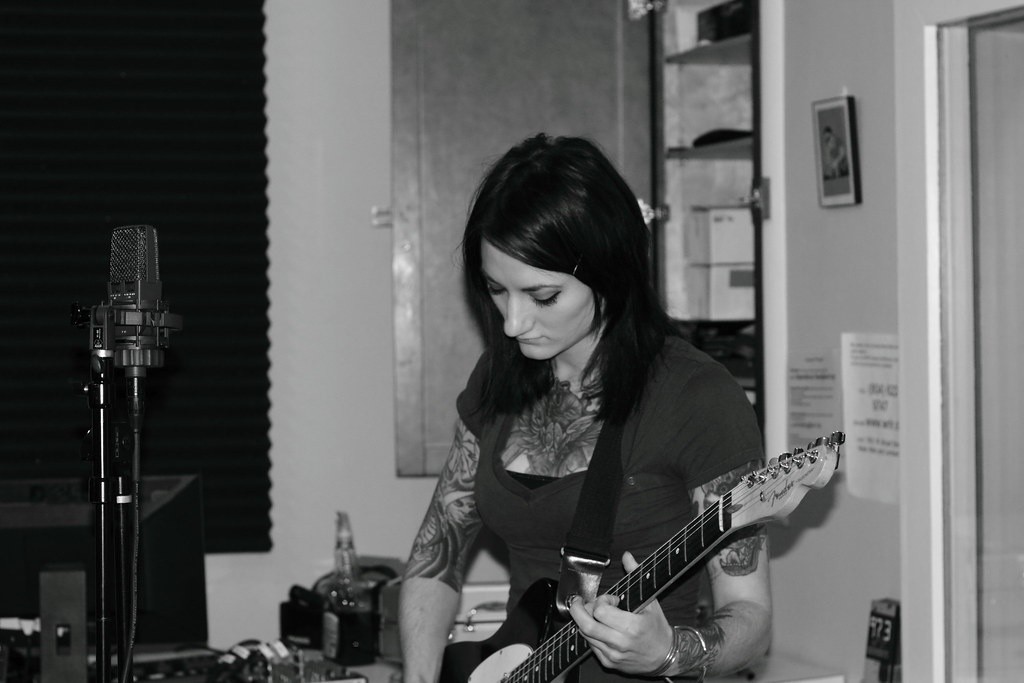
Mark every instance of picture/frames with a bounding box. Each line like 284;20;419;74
811;95;862;208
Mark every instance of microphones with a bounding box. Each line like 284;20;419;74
109;226;163;437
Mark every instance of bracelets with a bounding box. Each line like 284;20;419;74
649;625;707;683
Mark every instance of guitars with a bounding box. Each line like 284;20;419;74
436;428;848;682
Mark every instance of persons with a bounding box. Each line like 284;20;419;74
822;126;850;179
400;135;771;683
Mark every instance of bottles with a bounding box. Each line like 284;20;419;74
322;510;380;669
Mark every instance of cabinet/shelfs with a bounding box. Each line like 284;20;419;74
645;1;764;439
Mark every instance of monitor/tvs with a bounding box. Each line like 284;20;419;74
0;473;209;683
